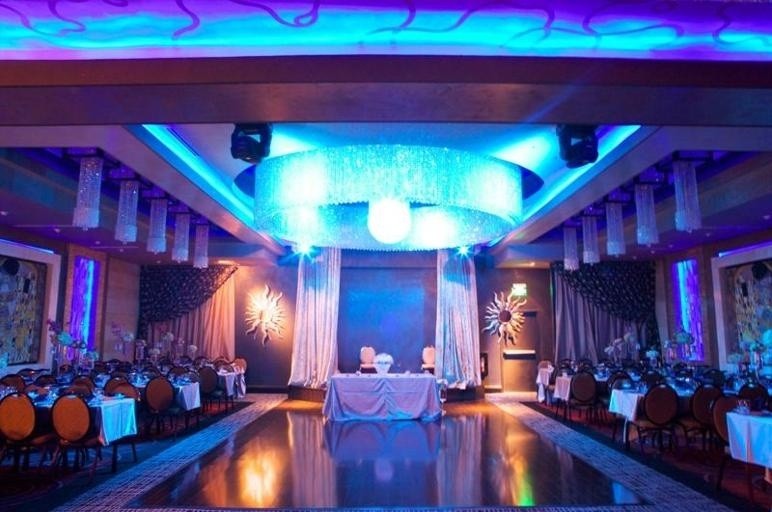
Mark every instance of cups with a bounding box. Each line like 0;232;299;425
734;399;751;415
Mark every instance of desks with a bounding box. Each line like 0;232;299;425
322;373;442;423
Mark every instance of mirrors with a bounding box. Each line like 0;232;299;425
244;284;286;347
480;288;527;345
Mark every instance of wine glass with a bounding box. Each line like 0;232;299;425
0;362;190;402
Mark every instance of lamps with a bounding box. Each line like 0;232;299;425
73;156;209;269
230;122;273;166
256;145;525;251
556;123;599;169
564;162;703;273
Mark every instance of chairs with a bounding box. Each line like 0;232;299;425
537;358;772;501
360;346;376;373
0;355;248;487
421;346;435;374
